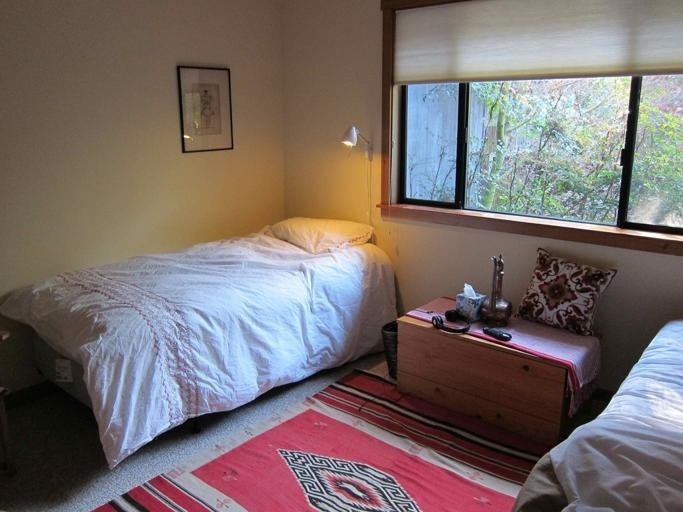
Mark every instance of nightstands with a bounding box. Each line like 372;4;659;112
397;300;603;446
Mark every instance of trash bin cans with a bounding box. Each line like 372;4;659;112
381;322;398;380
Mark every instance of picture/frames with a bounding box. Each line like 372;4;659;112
178;66;235;153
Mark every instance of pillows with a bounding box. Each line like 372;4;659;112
512;247;616;336
270;216;375;253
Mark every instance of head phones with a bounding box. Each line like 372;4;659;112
432;310;470;333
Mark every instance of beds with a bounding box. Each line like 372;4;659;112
2;219;395;469
526;321;682;512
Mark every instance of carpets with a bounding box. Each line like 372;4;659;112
95;371;545;512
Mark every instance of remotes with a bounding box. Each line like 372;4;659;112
483;326;512;341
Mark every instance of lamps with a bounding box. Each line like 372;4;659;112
340;124;375;161
